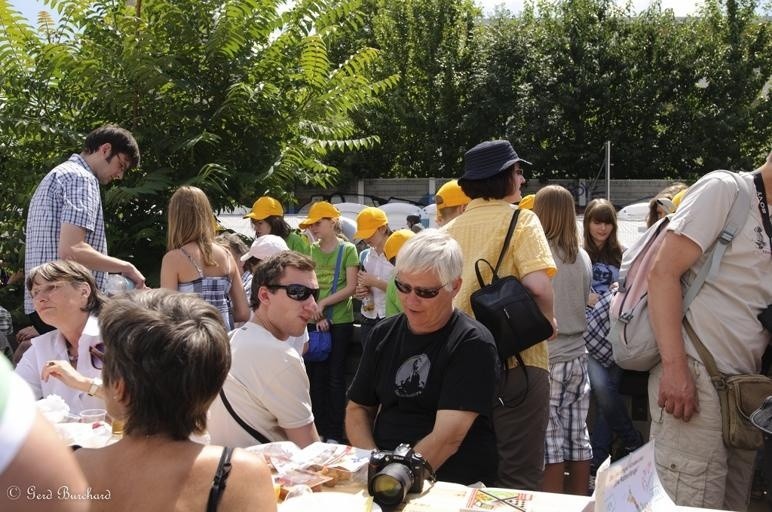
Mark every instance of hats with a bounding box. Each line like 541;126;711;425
457;140;534;186
243;180;535;262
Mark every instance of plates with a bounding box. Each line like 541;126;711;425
281;492;382;512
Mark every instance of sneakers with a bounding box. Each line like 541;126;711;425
623;430;644;457
589;465;598;495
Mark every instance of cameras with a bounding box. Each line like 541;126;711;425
366;443;435;512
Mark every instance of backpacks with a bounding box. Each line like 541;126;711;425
606;168;752;372
470;260;553;358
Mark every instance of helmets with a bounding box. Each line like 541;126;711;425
407;214;421;223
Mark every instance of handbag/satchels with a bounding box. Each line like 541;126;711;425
304;331;332;363
581;285;616;369
720;370;772;450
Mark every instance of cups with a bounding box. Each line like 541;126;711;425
81;409;108;445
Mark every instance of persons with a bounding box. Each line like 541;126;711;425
438;137;560;493
70;286;280;512
646;151;772;512
1;351;92;512
2;178;692;502
23;125;146;336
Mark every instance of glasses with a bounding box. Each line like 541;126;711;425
31;281;71;297
268;284;320;302
394;272;449;299
117;153;128;173
89;341;107;370
513;168;523;176
